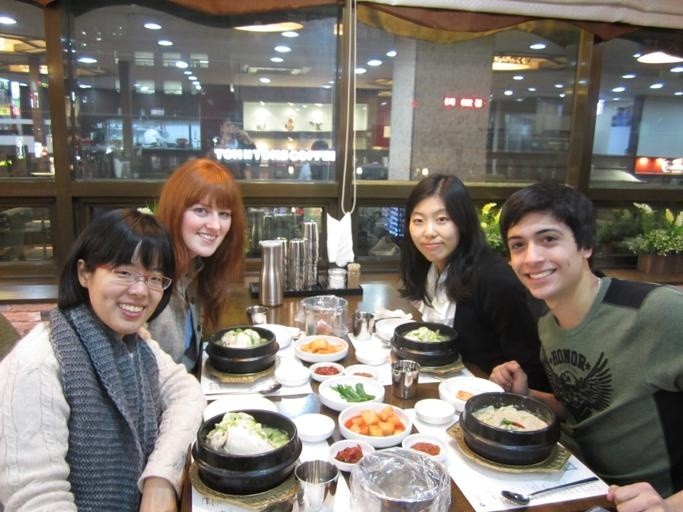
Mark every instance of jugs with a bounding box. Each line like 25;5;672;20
348;332;387;365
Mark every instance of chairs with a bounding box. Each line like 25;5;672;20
0;314;21;361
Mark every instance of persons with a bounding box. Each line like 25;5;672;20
610;106;630;126
144;157;247;373
206;118;260;180
488;181;683;512
297;139;330;180
0;208;207;512
357;151;389;180
143;124;167;146
395;172;553;396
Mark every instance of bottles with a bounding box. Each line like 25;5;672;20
327;268;346;289
70;135;121;180
259;239;281;307
347;263;362;289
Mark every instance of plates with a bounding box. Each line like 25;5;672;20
390;351;466;378
445;420;573;474
252;316;506;472
204;357;276;384
189;461;303;512
203;394;279;422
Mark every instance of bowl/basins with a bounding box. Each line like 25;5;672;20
310;362;346;381
350;446;451;512
389;323;461;367
461;392;559;466
414;398;457;426
375;321;401;340
439;375;507;414
293;413;336;444
318;374;387;413
339;401;414;449
205;327;279;374
275;365;310;386
189;408;303;493
297;296;346;334
345;364;381;379
402;434;448;463
329;439;375;471
294;336;351;361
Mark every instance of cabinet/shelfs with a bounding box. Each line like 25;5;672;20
141;146;201;171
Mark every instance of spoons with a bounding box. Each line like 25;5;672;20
501;477;600;503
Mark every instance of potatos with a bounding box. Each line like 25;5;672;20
347;405;405;438
301;339;344;355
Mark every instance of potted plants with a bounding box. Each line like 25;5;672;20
621;201;683;273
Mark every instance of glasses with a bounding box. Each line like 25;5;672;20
90;259;174;291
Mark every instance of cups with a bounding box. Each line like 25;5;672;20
247;305;272;330
274;220;319;292
294;460;339;512
390;361;421;399
